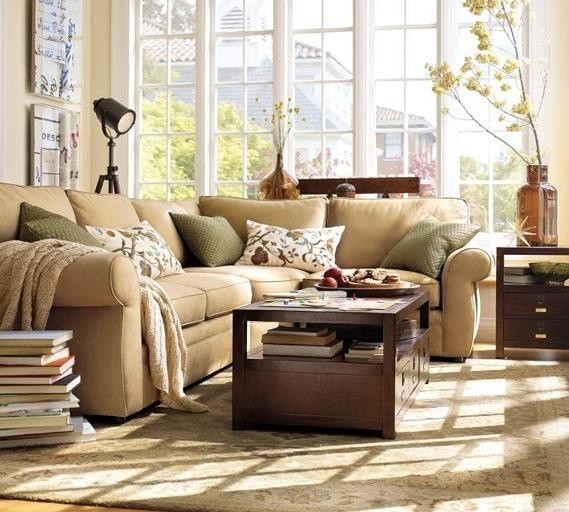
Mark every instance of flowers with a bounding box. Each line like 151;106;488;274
424;0;569;231
252;98;305;199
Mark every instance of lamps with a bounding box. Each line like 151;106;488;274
93;98;136;194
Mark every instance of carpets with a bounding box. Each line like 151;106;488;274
1;359;568;511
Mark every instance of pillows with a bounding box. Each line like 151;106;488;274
169;212;245;267
234;219;345;272
84;220;185;279
19;202;104;248
378;214;482;278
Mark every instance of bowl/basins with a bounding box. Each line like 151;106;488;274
528;261;569;286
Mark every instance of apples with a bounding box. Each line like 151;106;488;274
251;246;268;265
320;267;350;287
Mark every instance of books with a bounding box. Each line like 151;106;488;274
400;329;418;341
504;275;542;283
261;325;384;364
504;266;535;275
399;319;417;330
0;329;97;448
263;289;347;300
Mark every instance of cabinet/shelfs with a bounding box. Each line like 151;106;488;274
496;247;569;359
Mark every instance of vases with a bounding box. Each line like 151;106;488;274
516;165;557;246
256;153;301;200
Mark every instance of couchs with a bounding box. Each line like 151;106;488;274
0;183;494;421
